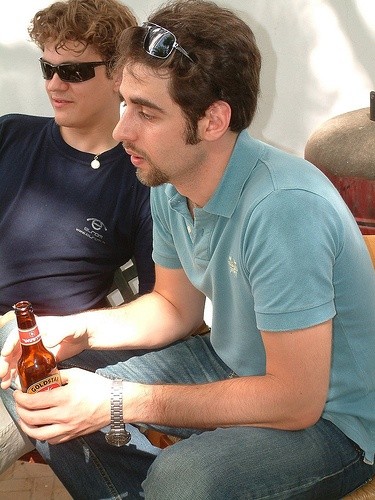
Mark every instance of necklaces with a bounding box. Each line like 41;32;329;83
61;134;121;169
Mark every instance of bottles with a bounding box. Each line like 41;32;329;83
12;300;63;427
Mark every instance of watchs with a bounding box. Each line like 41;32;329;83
106;379;131;447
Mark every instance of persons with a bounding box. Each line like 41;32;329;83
0;0;156;474
0;0;375;500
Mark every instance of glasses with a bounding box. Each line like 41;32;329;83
39;57;114;83
141;22;224;101
